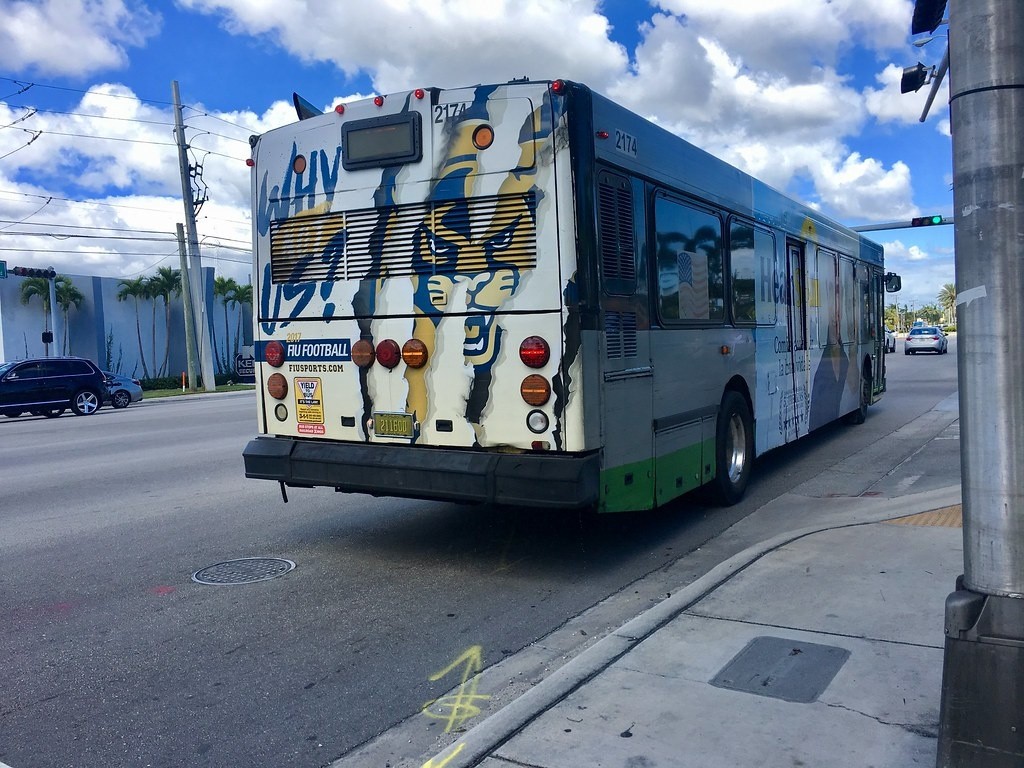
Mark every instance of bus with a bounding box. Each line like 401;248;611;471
240;81;903;520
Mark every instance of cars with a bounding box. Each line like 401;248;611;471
100;369;144;409
904;327;949;354
883;322;897;354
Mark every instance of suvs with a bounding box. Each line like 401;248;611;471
1;357;109;419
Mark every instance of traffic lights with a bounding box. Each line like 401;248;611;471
13;265;56;280
911;213;943;227
